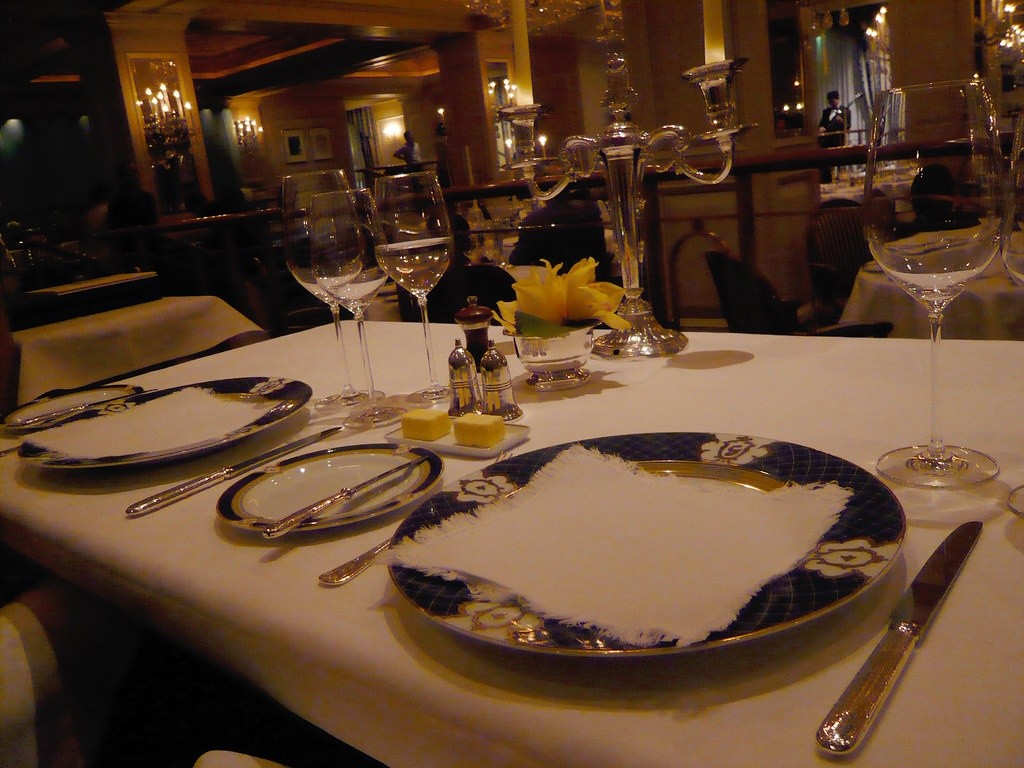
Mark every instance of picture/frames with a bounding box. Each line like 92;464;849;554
309;127;334;161
281;128;307;164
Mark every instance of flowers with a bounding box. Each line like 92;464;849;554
489;255;641;334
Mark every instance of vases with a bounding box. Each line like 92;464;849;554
502;323;597;390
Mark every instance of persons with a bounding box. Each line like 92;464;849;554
0;575;149;767
82;168;158;232
508;186;613;281
888;163;975;235
815;89;851;147
393;130;423;172
397;212;517;326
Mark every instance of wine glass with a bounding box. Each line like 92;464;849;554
374;172;454;404
863;80;1024;519
282;169;385;412
311;188;407;428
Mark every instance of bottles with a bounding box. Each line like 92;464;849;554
833;162;897;187
455;297;493;372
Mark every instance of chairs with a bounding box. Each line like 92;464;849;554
0;164;1024;768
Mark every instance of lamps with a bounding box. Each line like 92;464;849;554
136;85;192;154
866;7;891;55
488;80;517;123
232;115;265;158
973;1;1024;81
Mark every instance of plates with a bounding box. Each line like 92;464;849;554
17;377;313;468
388;431;908;658
216;444;445;531
5;384;144;430
384;416;532;457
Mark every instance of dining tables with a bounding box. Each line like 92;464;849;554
0;312;1024;768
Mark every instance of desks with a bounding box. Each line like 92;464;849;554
839;226;1024;337
14;295;268;411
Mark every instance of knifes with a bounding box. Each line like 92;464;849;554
125;427;343;515
21;389;158;425
816;521;983;754
263;455;429;539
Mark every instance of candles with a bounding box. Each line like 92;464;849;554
438;108;445;126
538;135;547;157
505;139;513;160
